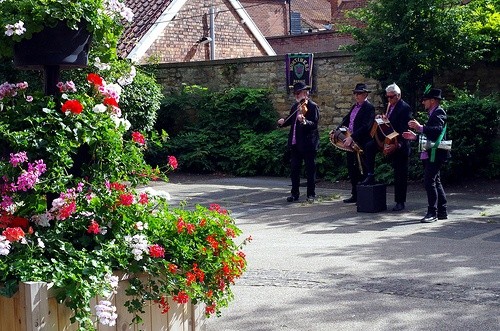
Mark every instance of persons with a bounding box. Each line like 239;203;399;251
375;82;415;210
276;83;320;202
402;89;448;222
328;83;375;203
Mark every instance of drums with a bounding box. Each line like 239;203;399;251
417;134;452;153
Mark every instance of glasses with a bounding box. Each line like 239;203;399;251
293;91;302;96
424;99;434;102
353;92;363;95
385;94;397;99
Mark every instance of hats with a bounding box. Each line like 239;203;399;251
292;83;311;93
424;89;443;100
351;83;372;93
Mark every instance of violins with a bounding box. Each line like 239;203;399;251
297;99;308;125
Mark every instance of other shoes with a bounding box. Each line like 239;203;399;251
343;195;357;203
392;203;405;211
287;195;299;203
420;215;438;223
437;214;447;220
308;197;314;203
